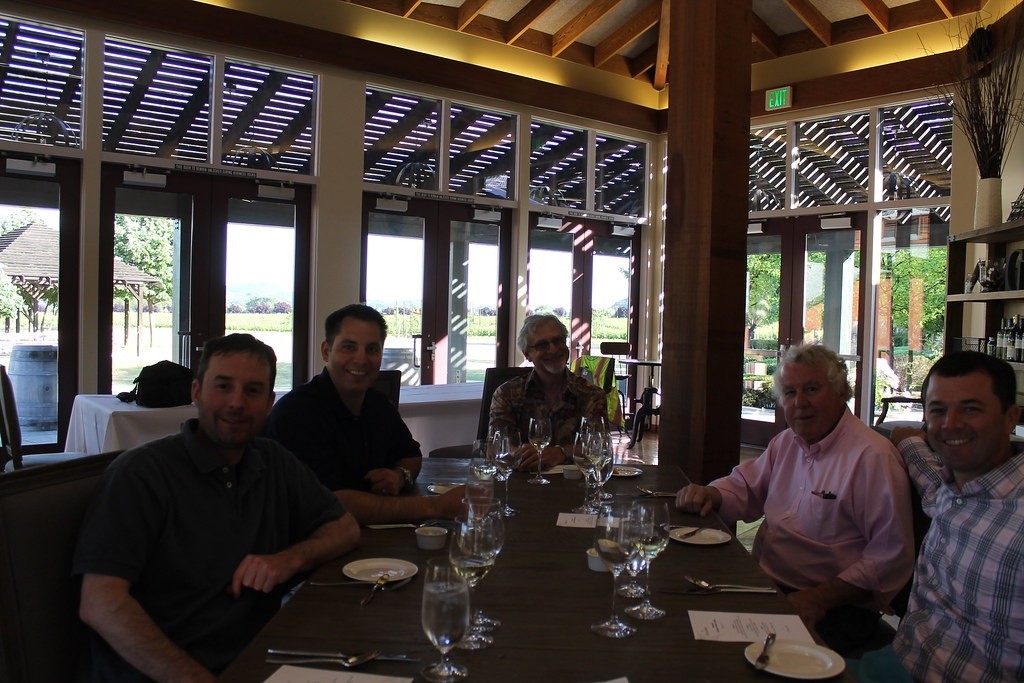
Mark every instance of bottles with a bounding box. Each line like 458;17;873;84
986;315;1024;362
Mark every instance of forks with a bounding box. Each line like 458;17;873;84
310;577;412;591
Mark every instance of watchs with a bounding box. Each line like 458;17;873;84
394;464;414;491
554;443;568;465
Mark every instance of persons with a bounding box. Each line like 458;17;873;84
841;349;1024;683
261;304;491;521
58;333;361;683
489;313;606;474
675;344;913;634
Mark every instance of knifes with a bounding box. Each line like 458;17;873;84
755;633;776;669
360;574;390;605
681;522;712;540
660;589;777;595
268;649;421;661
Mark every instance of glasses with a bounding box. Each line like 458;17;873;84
527;335;566;352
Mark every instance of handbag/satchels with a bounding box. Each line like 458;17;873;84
116;360;194;408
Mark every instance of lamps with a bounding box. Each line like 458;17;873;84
748;179;781;212
232;120;279;172
11;52;77;148
394;131;435;190
529;185;567;219
882;172;920;224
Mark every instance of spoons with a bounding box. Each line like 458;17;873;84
264;647;383;667
637;486;679;494
684;575;772;590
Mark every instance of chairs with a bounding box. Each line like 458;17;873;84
477;367;534;439
600;342;632;407
0;365;86;473
0;449;128;683
842;426;932;658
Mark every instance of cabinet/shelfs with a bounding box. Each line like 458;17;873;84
943;218;1024;442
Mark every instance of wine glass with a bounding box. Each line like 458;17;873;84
421;556;470;683
571;416;613;513
463;431;521;531
589;498;669;637
526;416;551;485
459;498;504;632
449;515;494;650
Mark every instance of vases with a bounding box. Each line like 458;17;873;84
974;178;1002;230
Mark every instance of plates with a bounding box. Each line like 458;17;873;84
343;557;418;581
670;527;732;545
427;483;466;495
612;465;642;476
745;639;846;679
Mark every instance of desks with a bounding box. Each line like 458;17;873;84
218;457;857;683
619;359;661;449
65;383;483;457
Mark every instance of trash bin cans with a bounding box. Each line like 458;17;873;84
8;345;58;430
383;348;418;387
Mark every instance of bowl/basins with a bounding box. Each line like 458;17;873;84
415;527;447;550
587;548;613;573
563;466;582;479
